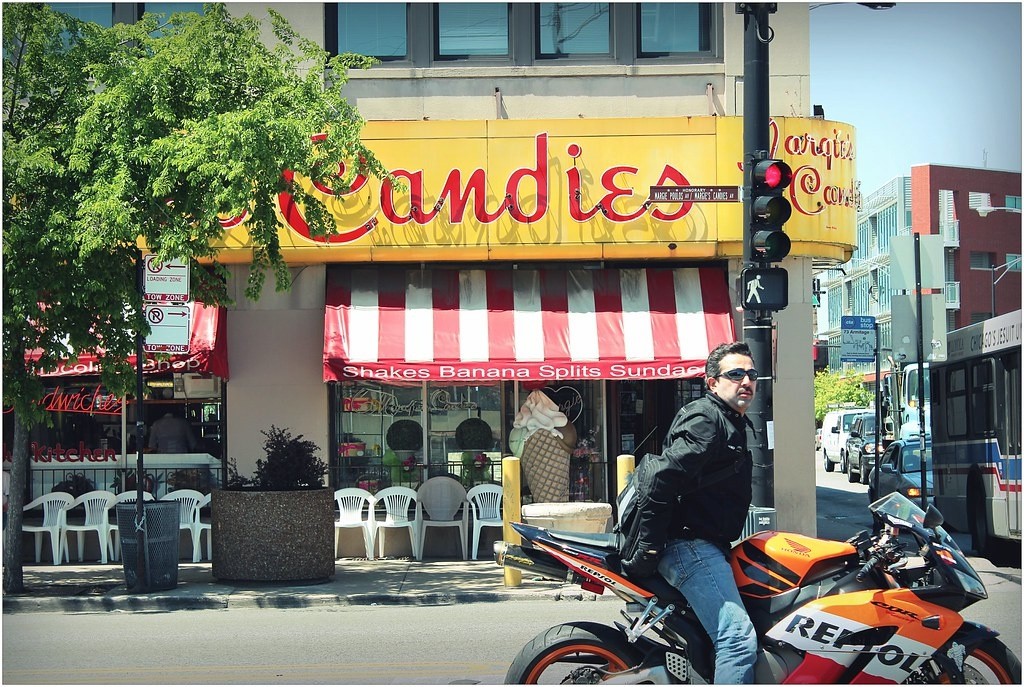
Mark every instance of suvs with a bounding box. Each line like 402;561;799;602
846;413;885;484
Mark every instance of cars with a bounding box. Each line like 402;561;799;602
868;437;934;511
816;429;823;450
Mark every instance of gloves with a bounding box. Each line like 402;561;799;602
620;549;661;581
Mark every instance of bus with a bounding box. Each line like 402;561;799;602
929;307;1022;563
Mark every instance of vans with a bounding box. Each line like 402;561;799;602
821;409;875;474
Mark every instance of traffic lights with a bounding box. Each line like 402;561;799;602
751;158;791;263
740;267;790;311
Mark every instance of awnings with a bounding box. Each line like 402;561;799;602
23;260;736;383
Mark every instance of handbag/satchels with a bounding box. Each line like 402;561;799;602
614;453;668;563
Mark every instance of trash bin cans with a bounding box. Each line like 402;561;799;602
117;500;181;595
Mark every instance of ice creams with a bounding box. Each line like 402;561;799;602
508;390;578;502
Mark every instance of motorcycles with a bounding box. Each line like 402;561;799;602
490;490;1022;685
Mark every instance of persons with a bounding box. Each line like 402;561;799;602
150;404;199;455
619;341;758;685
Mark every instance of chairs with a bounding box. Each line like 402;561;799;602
20;475;504;564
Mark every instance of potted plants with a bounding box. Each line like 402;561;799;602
455;417;493;460
386;419;423;463
212;424;335;582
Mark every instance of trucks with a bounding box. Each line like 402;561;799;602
881;363;931;433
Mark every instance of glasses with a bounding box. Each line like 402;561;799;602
714;368;758;381
